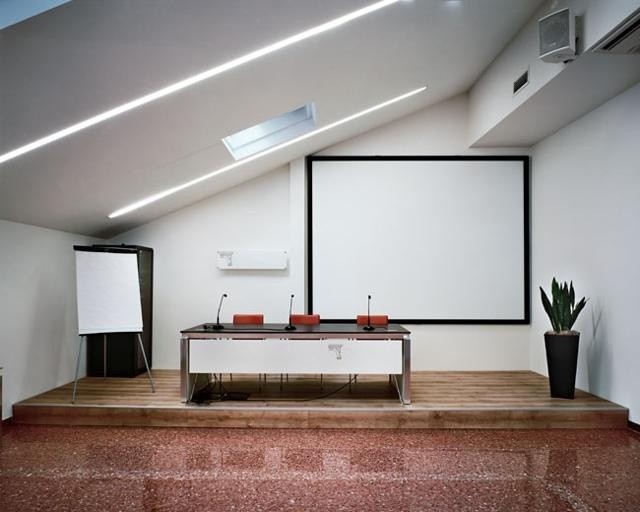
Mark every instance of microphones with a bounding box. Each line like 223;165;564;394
285;294;297;330
212;294;228;330
363;295;375;330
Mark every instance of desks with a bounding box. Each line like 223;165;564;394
180;323;411;404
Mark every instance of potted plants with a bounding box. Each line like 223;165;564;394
538;277;591;400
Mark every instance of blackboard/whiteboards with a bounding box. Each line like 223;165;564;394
73;246;144;337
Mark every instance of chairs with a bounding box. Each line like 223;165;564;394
220;315;266;392
280;315;324;393
348;315;395;393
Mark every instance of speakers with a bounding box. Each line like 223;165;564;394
537;8;578;62
